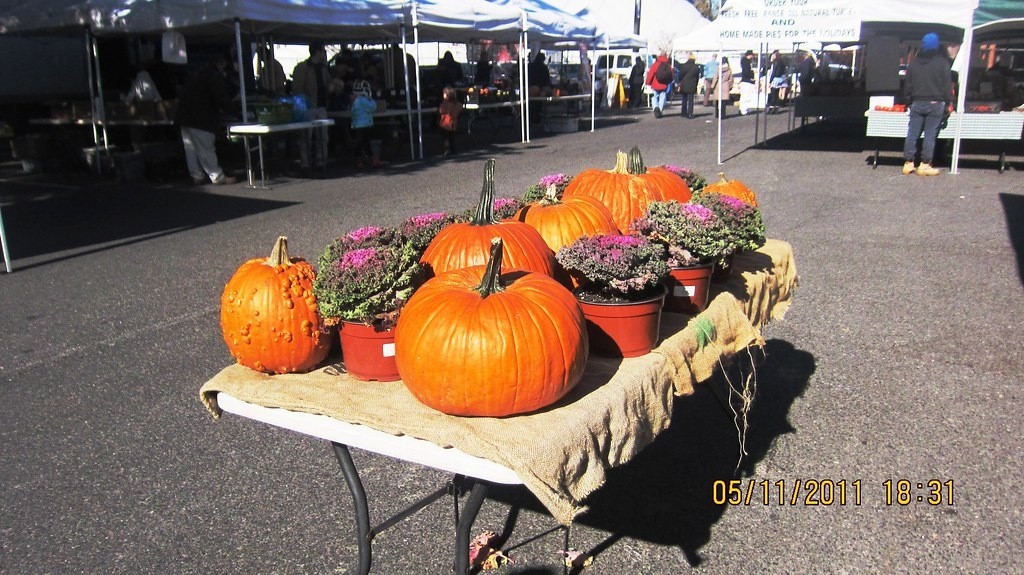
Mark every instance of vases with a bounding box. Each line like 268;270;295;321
702;249;735;284
573;285;669;358
337;318;403;383
664;261;715;314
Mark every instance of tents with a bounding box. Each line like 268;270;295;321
91;0;415;189
672;0;980;165
954;0;1024;174
488;0;604;132
541;25;650;107
0;0;139;174
259;0;531;143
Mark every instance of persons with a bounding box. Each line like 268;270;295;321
350;81;377;171
762;49;816;113
702;53;719;107
534;52;553;96
710;56;734;119
986;52;1011;74
435;51;464;85
335;57;354;72
739;50;756;115
678;52;699;118
628;56;645;107
646;51;674;118
902;32;954;175
174;53;237;185
473;51;493;87
293;41;331;169
255;45;286;97
437;86;463;157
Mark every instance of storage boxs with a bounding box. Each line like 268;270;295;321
543;115;579;133
869;96;895;110
893;104;905;112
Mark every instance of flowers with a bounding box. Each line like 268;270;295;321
313;165;768;333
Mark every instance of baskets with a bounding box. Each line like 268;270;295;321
250;103;295;125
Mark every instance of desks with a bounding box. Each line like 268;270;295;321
25;86;592;193
199;238;799;575
863;108;1024;174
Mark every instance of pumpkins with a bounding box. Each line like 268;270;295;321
218;236;335;373
394;237;589;417
419;146;760;292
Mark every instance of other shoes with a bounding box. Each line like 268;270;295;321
444;151;449;156
372;161;383;165
354;162;365;169
916;163;940;177
902;162;916;174
215;177;236;185
653;106;661;118
689;114;695;119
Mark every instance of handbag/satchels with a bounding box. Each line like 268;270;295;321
676;83;681;94
439;113;452;131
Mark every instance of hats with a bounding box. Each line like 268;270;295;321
353;80;371;97
919;33;939;51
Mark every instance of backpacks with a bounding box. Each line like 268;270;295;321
655;61;673;84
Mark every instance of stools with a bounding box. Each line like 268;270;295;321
82;145;117;171
